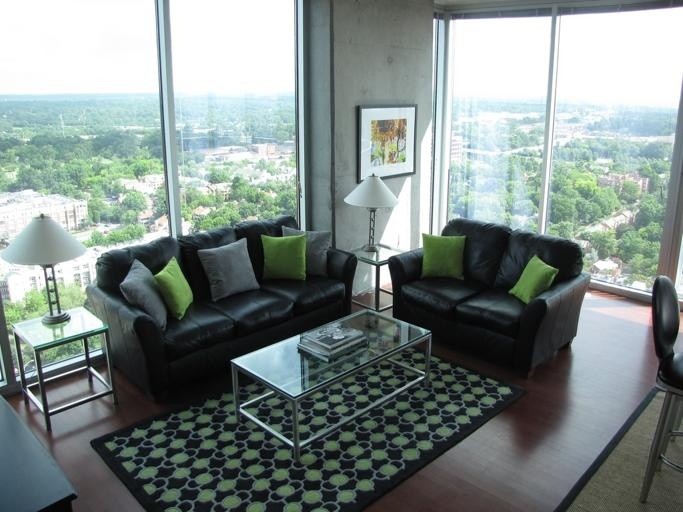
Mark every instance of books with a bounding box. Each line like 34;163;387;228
296;322;368;364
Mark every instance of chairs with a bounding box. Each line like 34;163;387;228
640;276;683;505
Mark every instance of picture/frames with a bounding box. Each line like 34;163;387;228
356;105;415;183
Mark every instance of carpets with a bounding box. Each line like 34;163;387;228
87;326;527;511
552;388;682;512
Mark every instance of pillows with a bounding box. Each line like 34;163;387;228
260;233;307;282
154;255;194;321
279;225;333;278
119;258;167;333
198;237;262;303
421;230;467;280
508;254;558;307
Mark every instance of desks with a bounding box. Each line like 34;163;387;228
10;308;119;432
0;395;79;512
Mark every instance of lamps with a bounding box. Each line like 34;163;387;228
1;214;86;326
343;174;397;251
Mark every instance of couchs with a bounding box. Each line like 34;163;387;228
85;214;358;394
388;217;590;377
